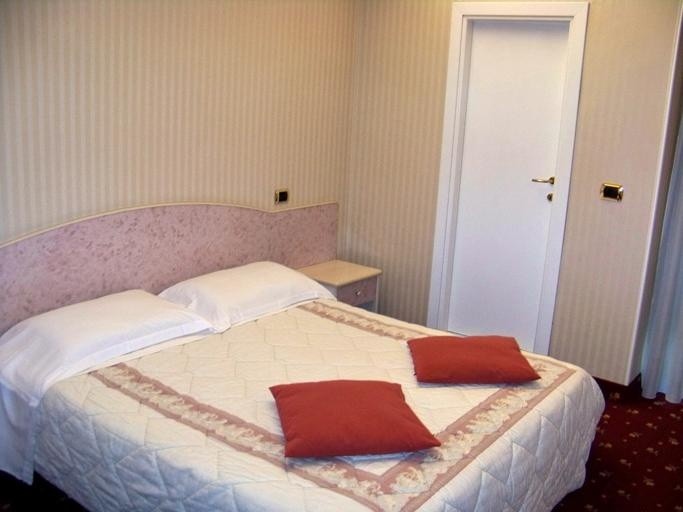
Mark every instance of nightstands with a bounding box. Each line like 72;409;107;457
294;259;381;314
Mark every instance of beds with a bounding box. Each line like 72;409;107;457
34;296;608;511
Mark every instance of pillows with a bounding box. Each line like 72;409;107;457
408;335;542;384
269;380;440;458
159;260;337;333
2;290;219;407
